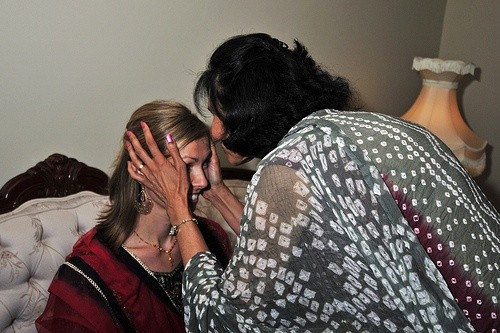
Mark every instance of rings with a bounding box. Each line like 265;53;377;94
139;163;144;172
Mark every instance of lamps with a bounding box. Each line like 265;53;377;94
399;57;489;178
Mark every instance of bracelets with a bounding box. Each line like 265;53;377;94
169;219;198;236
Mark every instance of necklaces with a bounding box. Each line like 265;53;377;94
134;231;177;268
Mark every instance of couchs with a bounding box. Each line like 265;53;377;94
0;154;262;333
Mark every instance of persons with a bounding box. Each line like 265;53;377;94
125;32;500;333
35;100;234;333
